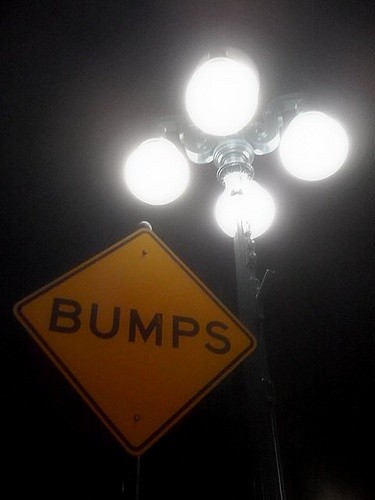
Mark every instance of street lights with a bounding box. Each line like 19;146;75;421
124;46;348;500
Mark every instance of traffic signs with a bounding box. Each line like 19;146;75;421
14;228;255;455
